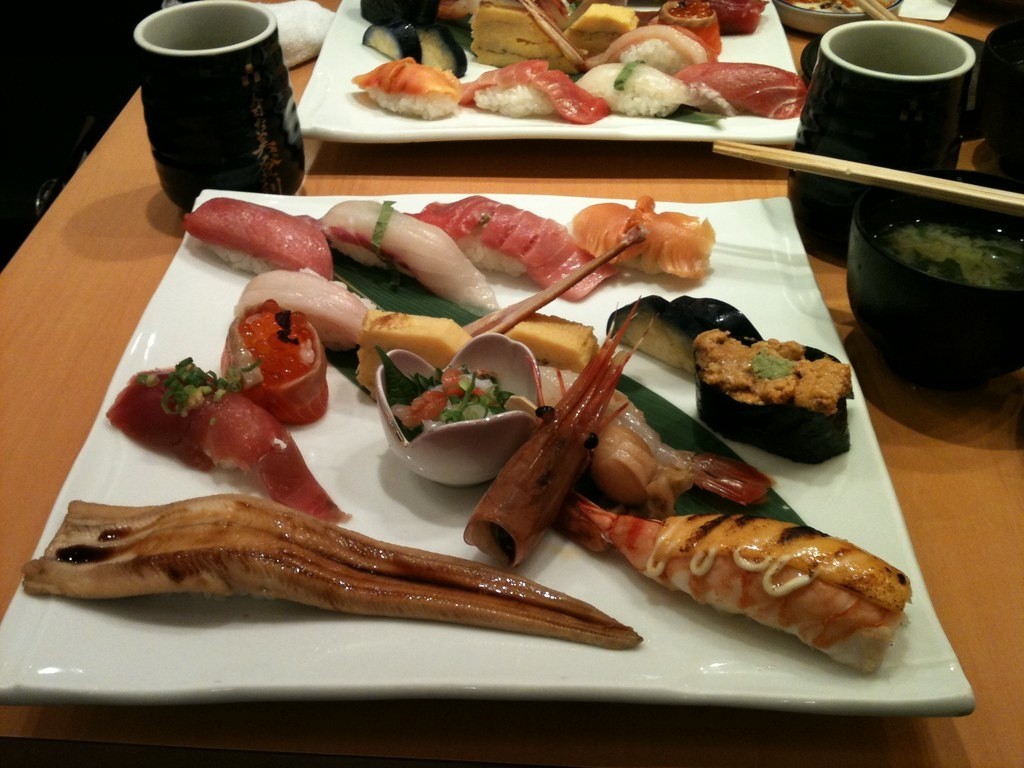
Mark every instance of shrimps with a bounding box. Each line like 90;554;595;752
460;299;914;677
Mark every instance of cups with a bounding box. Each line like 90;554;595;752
787;20;975;247
134;2;304;213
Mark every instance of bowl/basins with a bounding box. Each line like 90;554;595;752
774;0;904;33
976;18;1024;178
846;170;1023;392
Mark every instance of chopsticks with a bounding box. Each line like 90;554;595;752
713;138;1024;218
852;0;901;21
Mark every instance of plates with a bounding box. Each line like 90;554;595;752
0;190;976;719
800;31;987;125
298;1;803;145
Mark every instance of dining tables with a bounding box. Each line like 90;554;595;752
0;0;1024;768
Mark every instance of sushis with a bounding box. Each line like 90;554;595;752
348;0;808;122
20;194;915;673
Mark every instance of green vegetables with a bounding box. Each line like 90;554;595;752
874;221;1024;291
